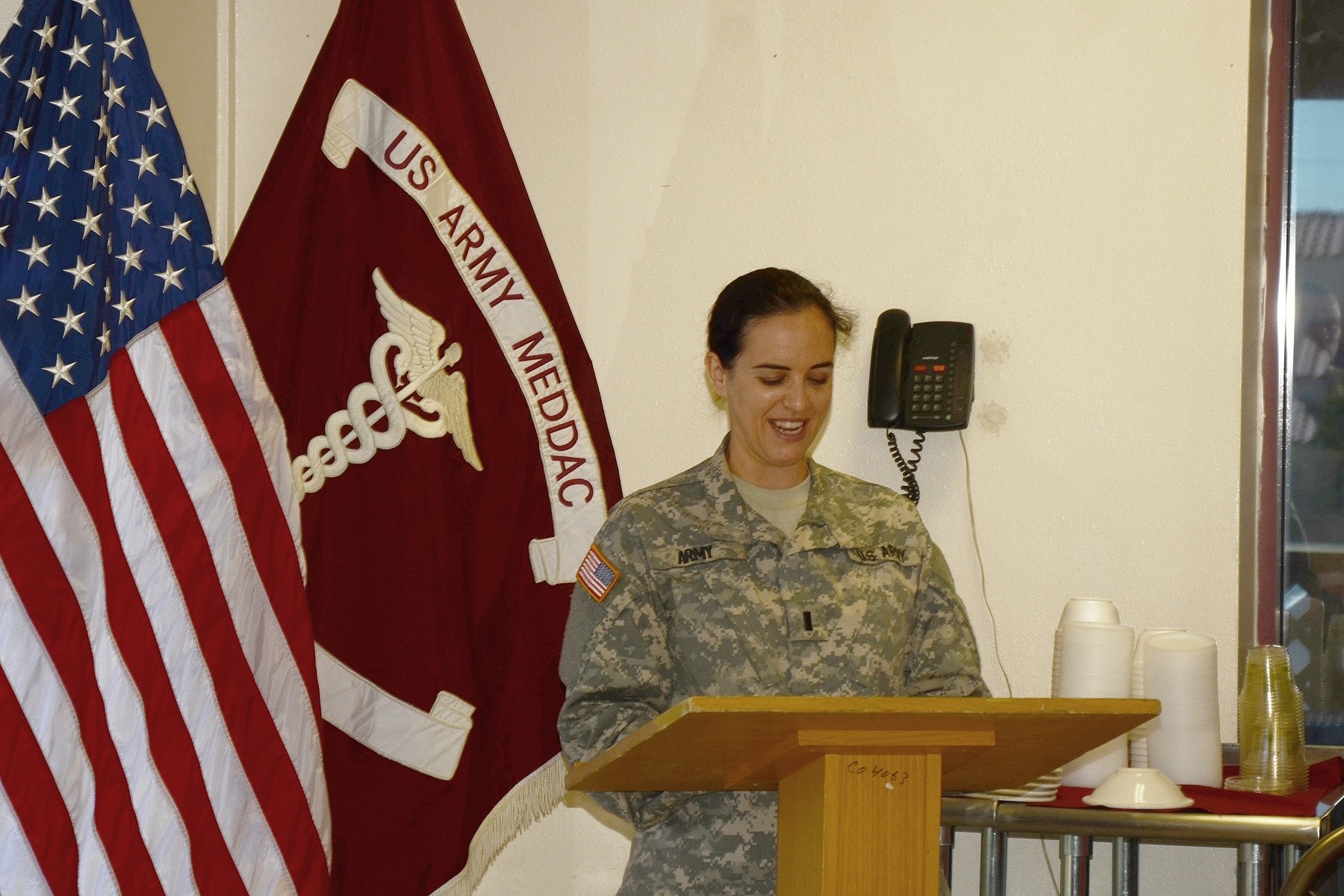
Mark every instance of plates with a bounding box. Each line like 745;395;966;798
964;762;1062;803
1141;633;1223;788
1058;622;1135;791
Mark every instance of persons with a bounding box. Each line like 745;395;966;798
555;270;992;895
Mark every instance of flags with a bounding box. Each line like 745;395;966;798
215;0;623;896
0;0;338;896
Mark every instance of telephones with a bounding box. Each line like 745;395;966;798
867;309;974;432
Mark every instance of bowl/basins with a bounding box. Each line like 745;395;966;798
1130;626;1186;768
1238;642;1309;792
1081;769;1196;810
1050;597;1121;701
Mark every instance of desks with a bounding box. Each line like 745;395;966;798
936;743;1344;896
